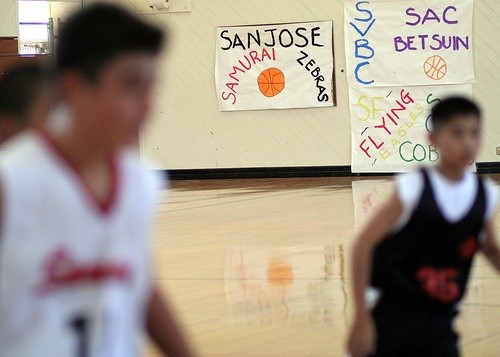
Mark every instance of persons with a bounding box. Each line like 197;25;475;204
1;57;55;146
1;2;197;357
345;95;500;357
